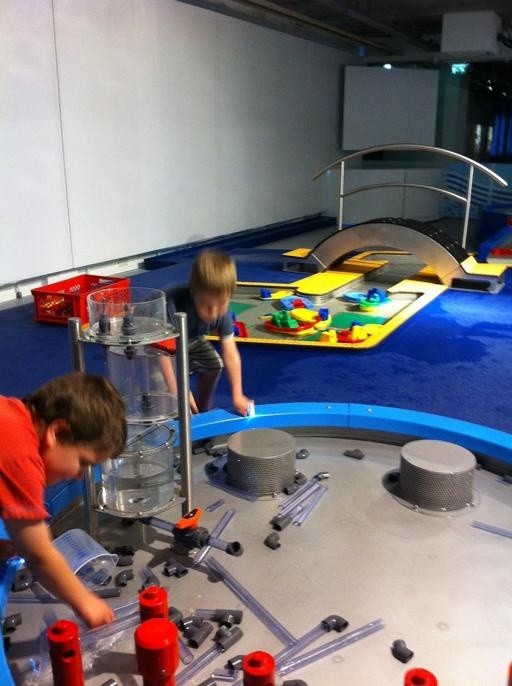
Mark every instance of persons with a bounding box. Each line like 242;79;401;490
135;248;253;419
0;370;128;630
